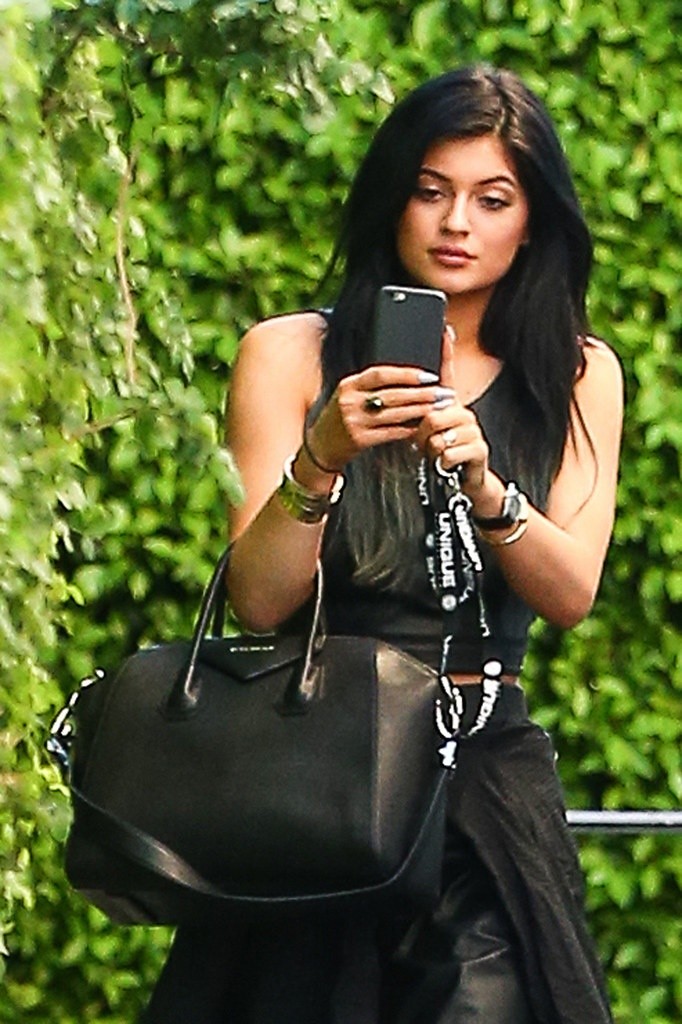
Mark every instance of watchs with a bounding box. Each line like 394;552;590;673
466;479;523;532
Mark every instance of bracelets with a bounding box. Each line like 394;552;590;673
276;456;334;525
302;433;341;475
469;485;529;547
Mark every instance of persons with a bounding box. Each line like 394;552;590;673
137;64;625;1024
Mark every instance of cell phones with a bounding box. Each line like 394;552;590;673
364;284;446;426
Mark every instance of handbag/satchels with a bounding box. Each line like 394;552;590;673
45;532;464;921
